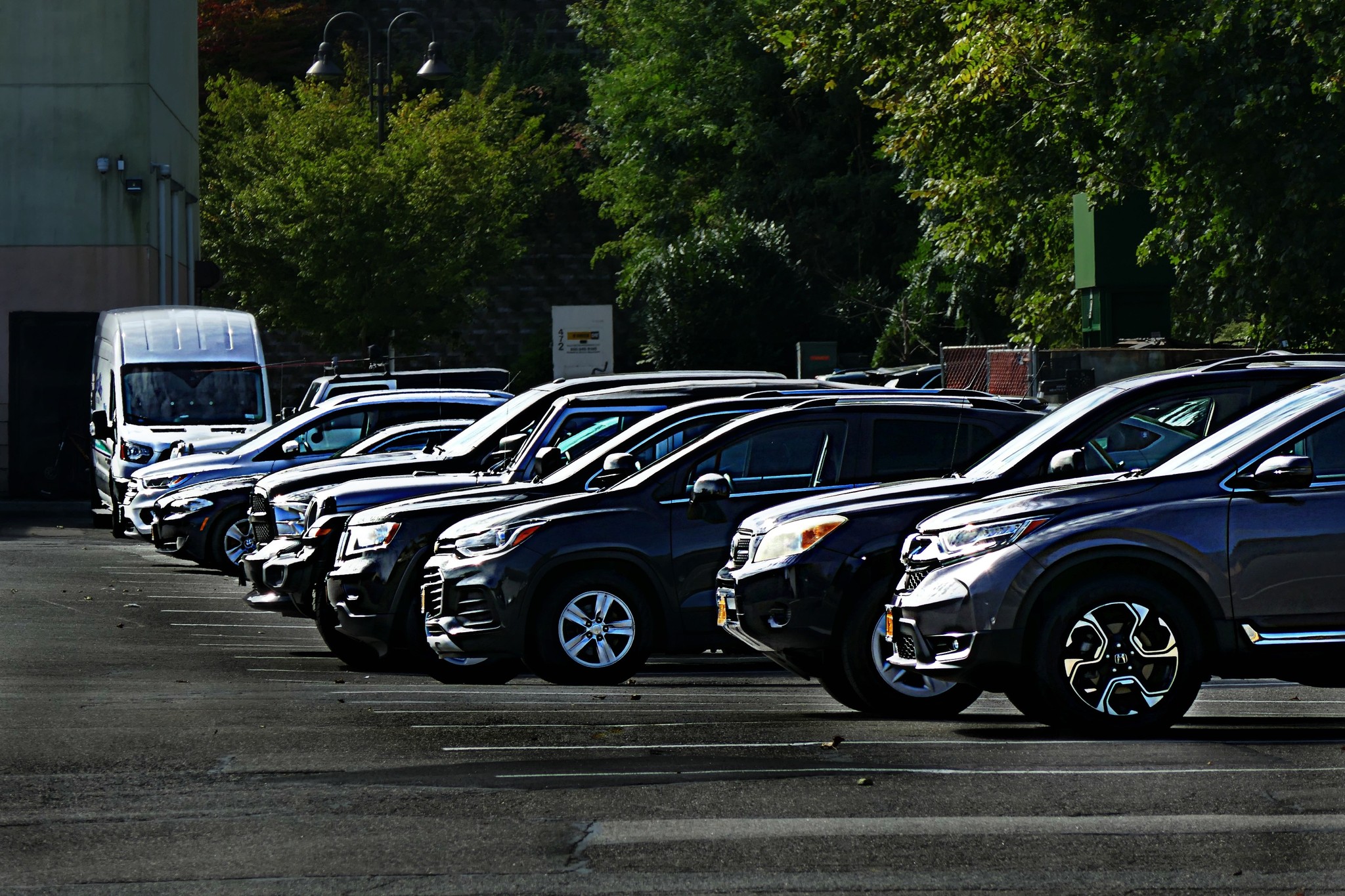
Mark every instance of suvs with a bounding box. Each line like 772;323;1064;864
325;373;833;671
711;349;1345;715
169;367;509;459
883;370;1344;740
150;418;481;578
417;394;1132;684
1036;378;1071;403
882;360;997;392
244;370;787;633
123;387;518;534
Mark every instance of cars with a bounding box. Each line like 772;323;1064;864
150;388;528;577
1044;402;1202;473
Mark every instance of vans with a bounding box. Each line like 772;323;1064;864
89;305;273;536
